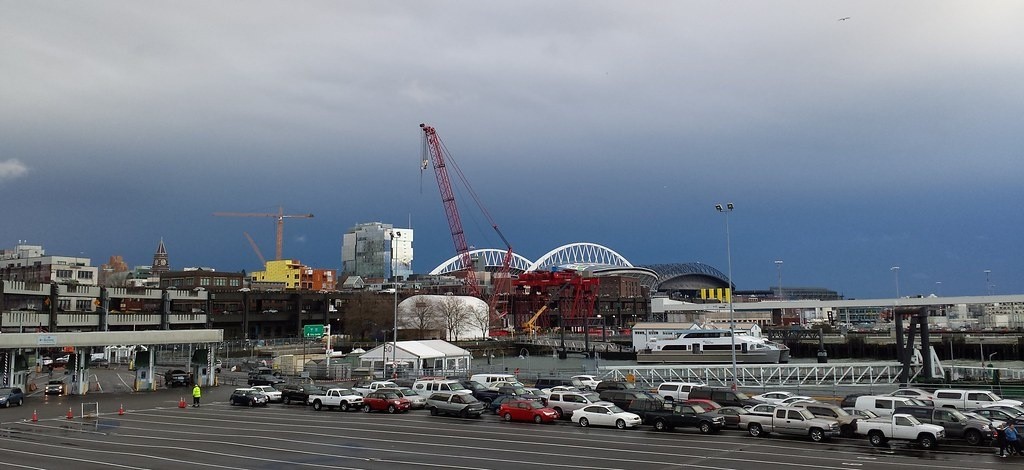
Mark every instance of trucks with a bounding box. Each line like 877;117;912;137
470;374;524;388
412;380;474;400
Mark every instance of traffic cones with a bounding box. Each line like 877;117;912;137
128;361;133;371
119;404;124;415
178;397;186;408
67;406;73;419
95;384;99;391
32;409;37;422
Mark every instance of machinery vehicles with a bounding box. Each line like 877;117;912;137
521;282;571;336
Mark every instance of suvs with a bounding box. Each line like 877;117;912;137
282;383;327;405
165;369;190;386
91;359;109;368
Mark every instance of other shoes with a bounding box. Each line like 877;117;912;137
1011;452;1024;456
1000;455;1007;458
192;404;200;407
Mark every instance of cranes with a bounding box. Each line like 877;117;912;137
210;206;315;260
420;123;513;338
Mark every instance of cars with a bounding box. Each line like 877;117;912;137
230;366;1024;450
0;387;24;408
45;379;65;395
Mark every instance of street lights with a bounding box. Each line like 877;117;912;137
519;347;529;369
715;202;737;390
491;348;504;373
891;266;900;298
774;260;785;326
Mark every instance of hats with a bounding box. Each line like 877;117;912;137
195;385;198;387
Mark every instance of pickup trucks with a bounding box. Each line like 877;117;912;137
352;381;399;397
737;406;843;442
309;388;364;412
643;403;726;434
854;414;946;449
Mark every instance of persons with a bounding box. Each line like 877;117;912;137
192;385;201;407
48;365;53;376
165;370;171;385
998;425;1006;458
1005;423;1019;456
